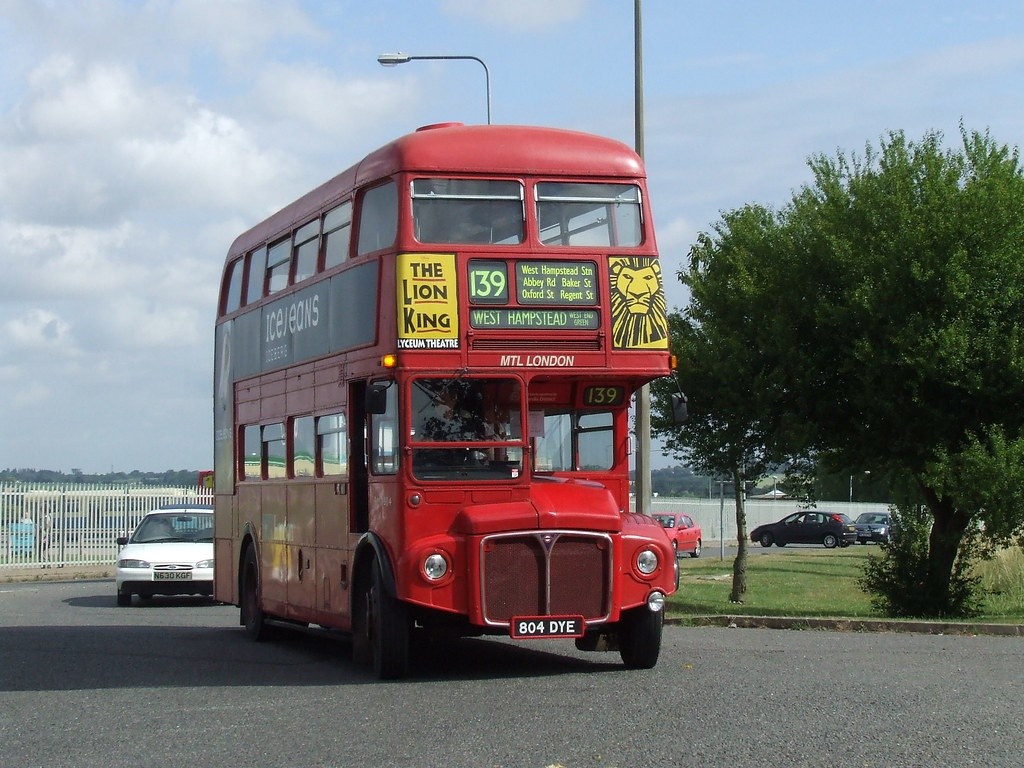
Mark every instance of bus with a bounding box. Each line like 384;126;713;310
213;122;681;681
23;488;196;544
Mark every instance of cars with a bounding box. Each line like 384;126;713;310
750;510;858;550
115;504;233;606
852;512;899;545
651;513;702;559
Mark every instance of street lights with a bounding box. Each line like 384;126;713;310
850;470;870;503
378;49;491;127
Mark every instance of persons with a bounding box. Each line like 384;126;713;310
418;380;487;469
34;503;53;569
21;512;33;524
